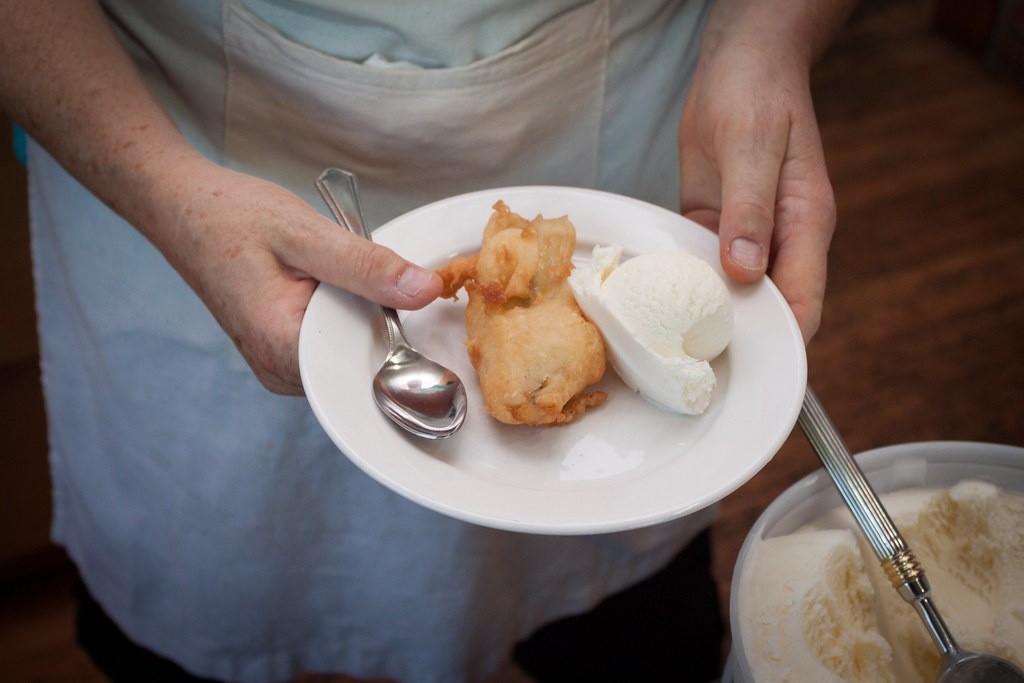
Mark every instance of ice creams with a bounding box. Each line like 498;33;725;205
571;243;735;416
738;479;1024;683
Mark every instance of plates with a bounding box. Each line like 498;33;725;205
299;187;808;534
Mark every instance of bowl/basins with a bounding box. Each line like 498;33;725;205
721;440;1024;682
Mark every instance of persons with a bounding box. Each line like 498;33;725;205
0;0;855;683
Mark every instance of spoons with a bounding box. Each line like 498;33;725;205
796;387;1024;683
314;167;466;439
